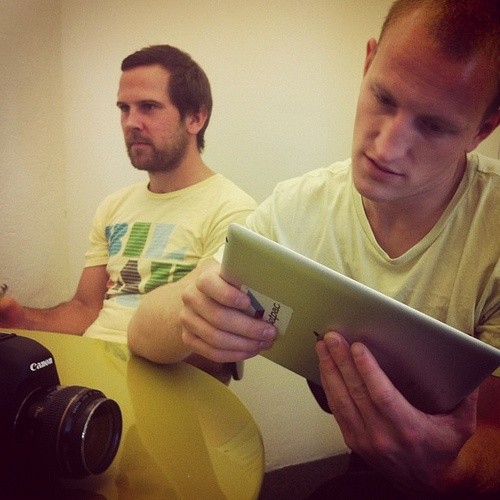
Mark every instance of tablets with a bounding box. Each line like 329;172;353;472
218;224;500;414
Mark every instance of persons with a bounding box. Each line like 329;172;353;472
124;1;499;500
0;42;265;389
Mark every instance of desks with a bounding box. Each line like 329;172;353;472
1;326;266;499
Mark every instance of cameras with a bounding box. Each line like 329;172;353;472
0;332;123;500
